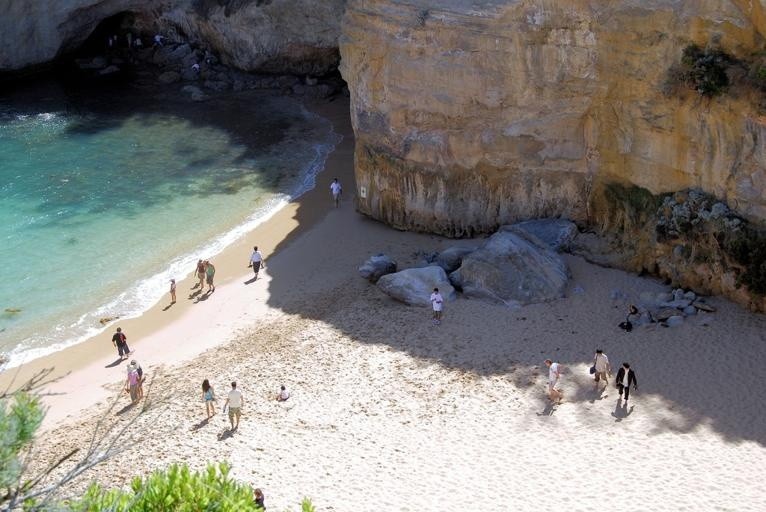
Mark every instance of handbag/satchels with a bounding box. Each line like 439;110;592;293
590;366;596;374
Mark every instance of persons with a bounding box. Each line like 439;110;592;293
616;362;637;404
330;178;343;208
627;303;637;316
248;246;264;278
202;379;216;419
430;288;444;324
169;278;176;304
592;349;611;386
251;488;266;511
223;381;243;430
204;261;215;291
126;359;143;402
275;386;290;401
195;260;205;289
544;359;563;402
112;327;130;360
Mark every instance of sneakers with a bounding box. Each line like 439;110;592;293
433;318;440;324
